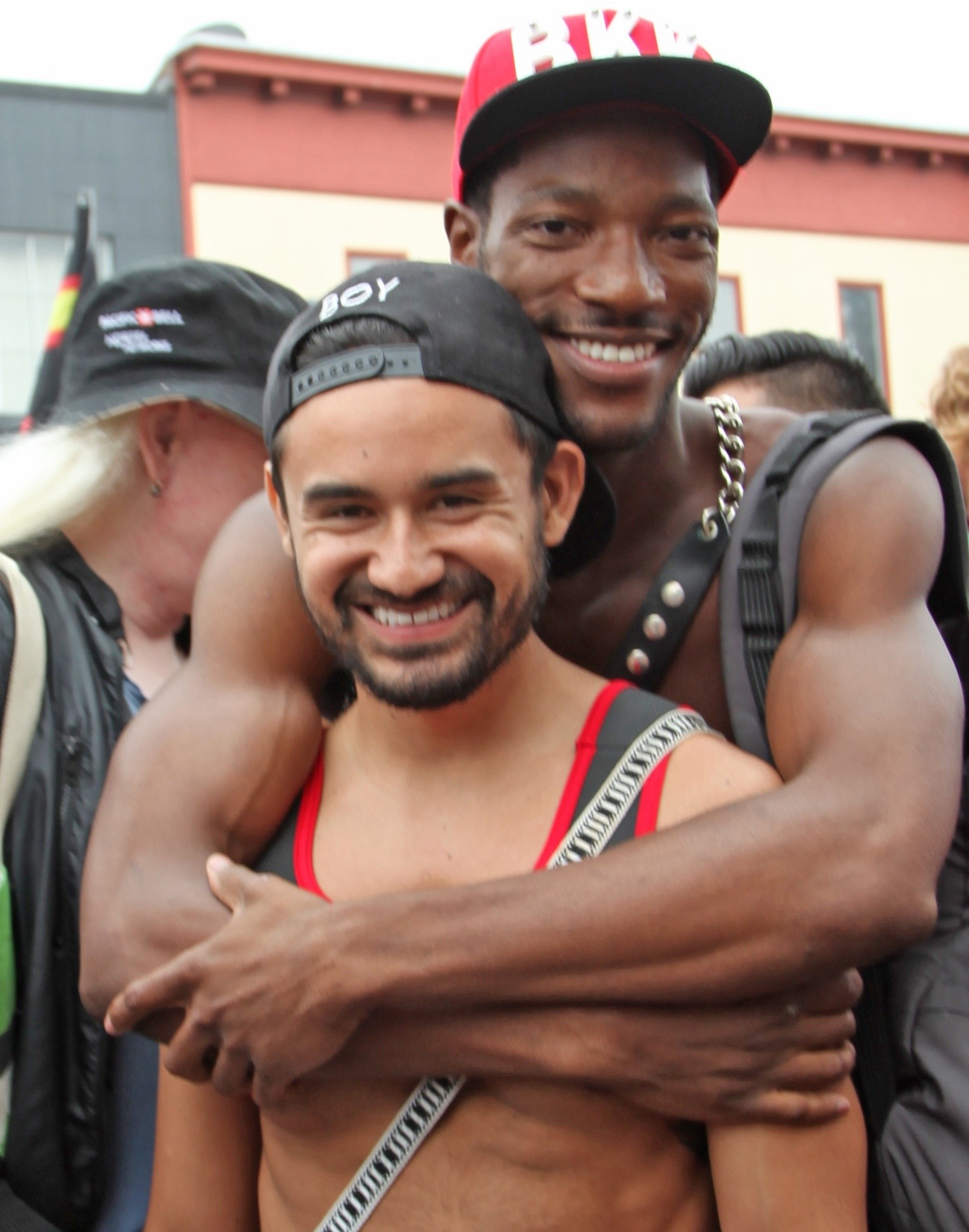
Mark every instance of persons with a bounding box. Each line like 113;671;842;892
1;11;969;1231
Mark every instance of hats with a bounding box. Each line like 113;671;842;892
263;260;615;580
49;261;308;436
453;10;774;209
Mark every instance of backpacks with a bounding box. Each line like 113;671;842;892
721;409;968;1231
